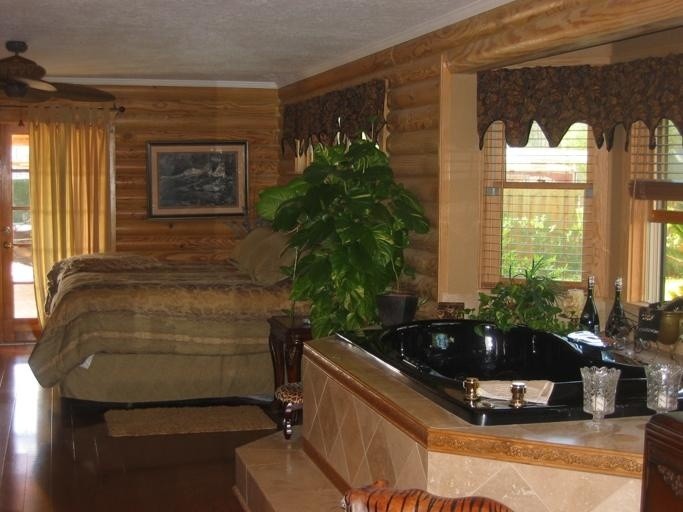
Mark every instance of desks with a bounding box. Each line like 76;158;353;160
270;315;310;423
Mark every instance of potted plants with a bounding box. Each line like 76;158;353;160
253;138;431;339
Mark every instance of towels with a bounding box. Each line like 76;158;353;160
473;380;555;405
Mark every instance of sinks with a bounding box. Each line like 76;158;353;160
334;320;683;424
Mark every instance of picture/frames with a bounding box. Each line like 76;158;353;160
143;138;251;222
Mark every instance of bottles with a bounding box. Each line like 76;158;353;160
604;276;631;341
579;275;599;335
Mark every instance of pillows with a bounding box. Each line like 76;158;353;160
227;228;312;287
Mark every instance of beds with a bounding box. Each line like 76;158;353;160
58;246;334;405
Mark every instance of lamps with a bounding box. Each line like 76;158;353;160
0;42;117;103
